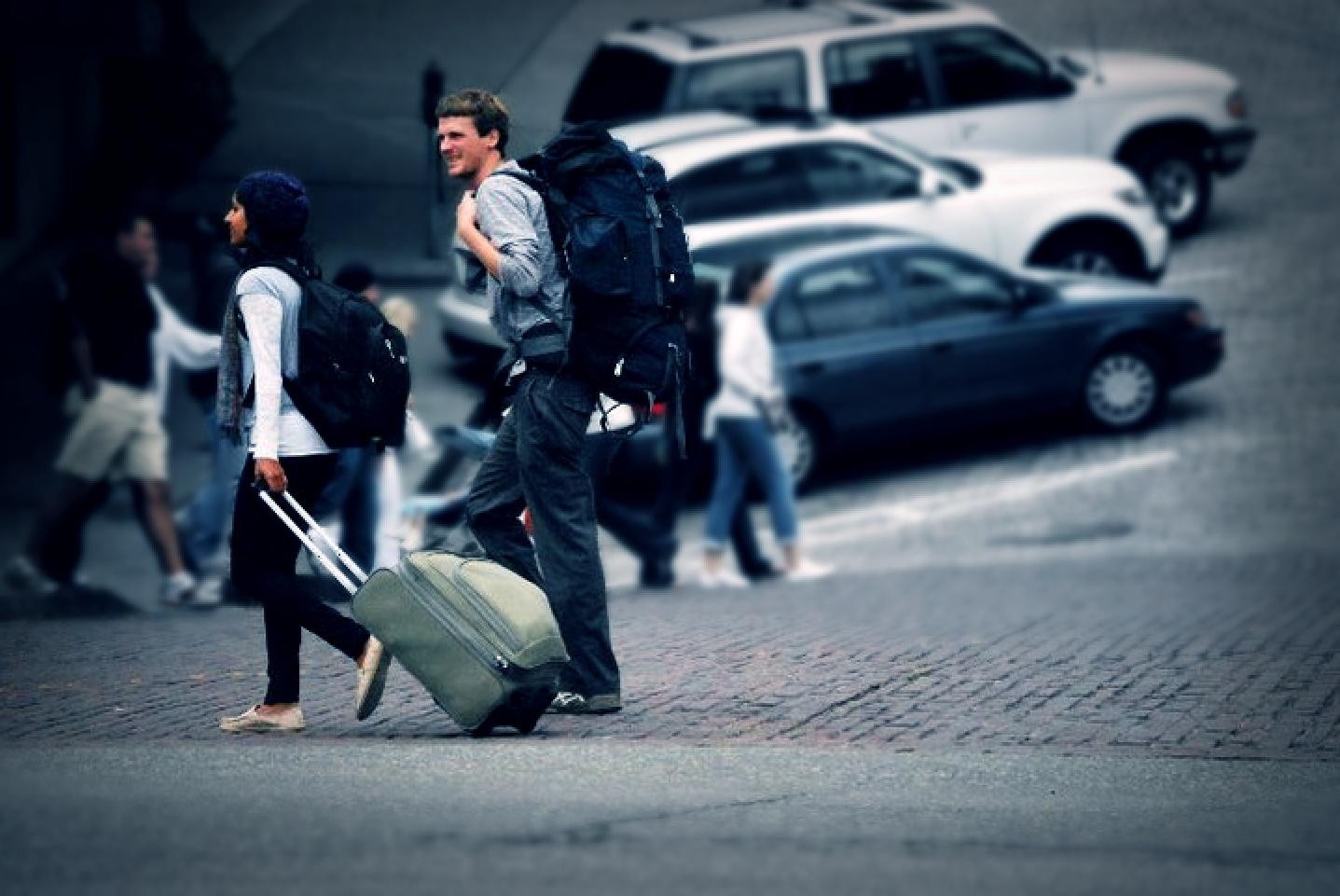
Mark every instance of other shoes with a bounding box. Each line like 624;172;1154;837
787;565;834;580
640;567;672;587
742;560;773;579
221;704;305;732
544;691;622;713
0;553;58;594
355;635;392;718
163;570;194;603
191;578;222;605
701;570;746;588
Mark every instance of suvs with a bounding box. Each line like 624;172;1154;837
548;0;1261;242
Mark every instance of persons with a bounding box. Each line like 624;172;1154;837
13;216;223;606
692;258;834;590
438;88;621;712
218;172;414;731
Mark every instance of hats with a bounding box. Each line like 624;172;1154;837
235;170;312;251
334;263;373;294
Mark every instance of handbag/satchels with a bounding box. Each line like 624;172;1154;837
762;400;787;431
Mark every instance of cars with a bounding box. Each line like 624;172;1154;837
433;105;1174;403
581;219;1229;511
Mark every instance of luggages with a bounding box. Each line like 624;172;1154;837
250;479;570;734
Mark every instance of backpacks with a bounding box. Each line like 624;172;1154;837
235;260;411;449
476;120;694;437
